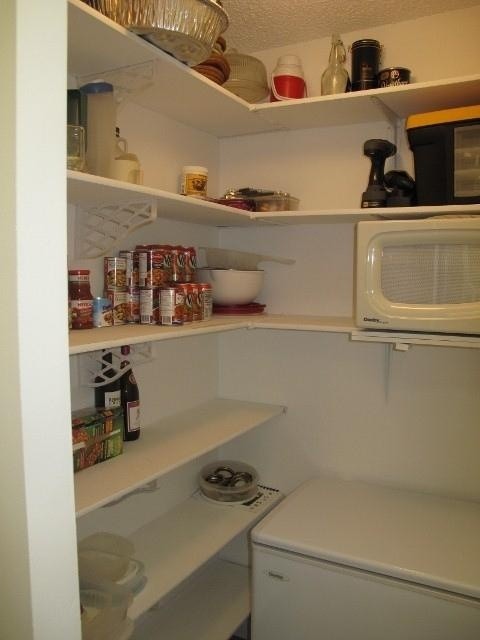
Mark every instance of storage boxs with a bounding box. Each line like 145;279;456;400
405;106;479;202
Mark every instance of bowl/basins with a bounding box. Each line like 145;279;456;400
90;0;231;69
194;267;263;306
198;458;259;504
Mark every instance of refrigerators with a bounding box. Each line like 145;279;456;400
248;477;479;640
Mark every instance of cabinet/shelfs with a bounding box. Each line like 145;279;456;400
64;3;255;358
255;70;480;341
68;394;298;636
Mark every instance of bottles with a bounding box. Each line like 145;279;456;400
346;38;382;90
68;265;94;329
80;81;115;177
319;30;349;96
119;346;142;440
94;347;122;409
269;53;309;104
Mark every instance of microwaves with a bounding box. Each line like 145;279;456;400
351;214;480;337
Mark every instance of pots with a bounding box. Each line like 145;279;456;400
202;247;297;271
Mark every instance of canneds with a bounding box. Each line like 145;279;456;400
68;244;213;330
377;67;410;88
181;165;209;200
350;38;381;92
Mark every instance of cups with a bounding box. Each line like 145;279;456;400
114;159;140;184
115;127;127;160
66;124;84;170
